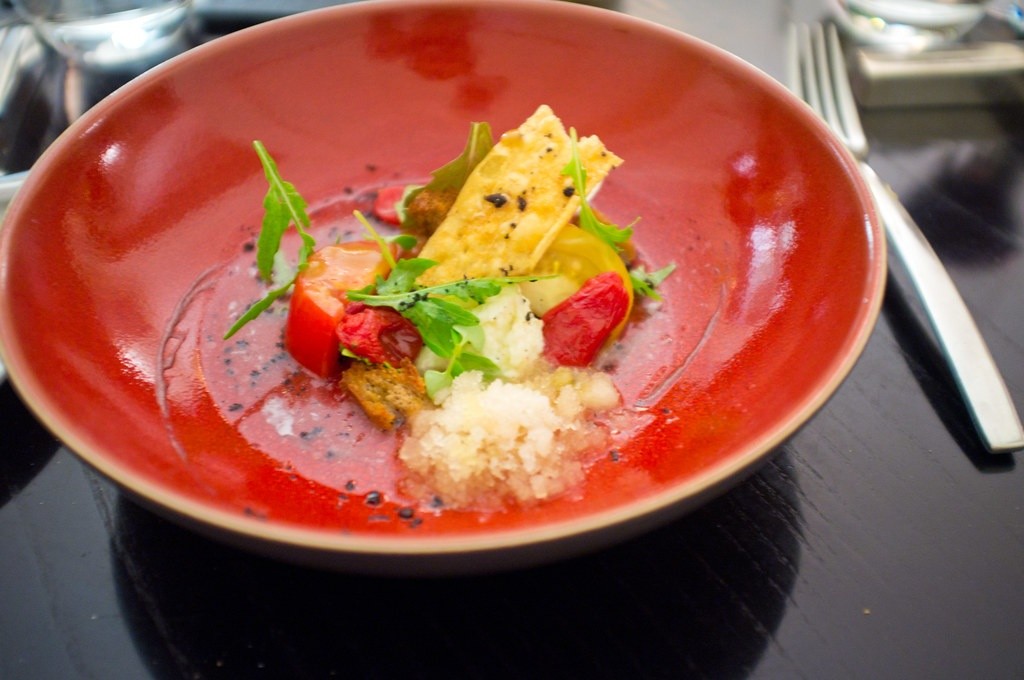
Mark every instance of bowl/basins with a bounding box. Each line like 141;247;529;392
1;0;886;572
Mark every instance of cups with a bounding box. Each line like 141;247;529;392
11;0;192;74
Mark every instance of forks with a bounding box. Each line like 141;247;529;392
785;20;1023;456
